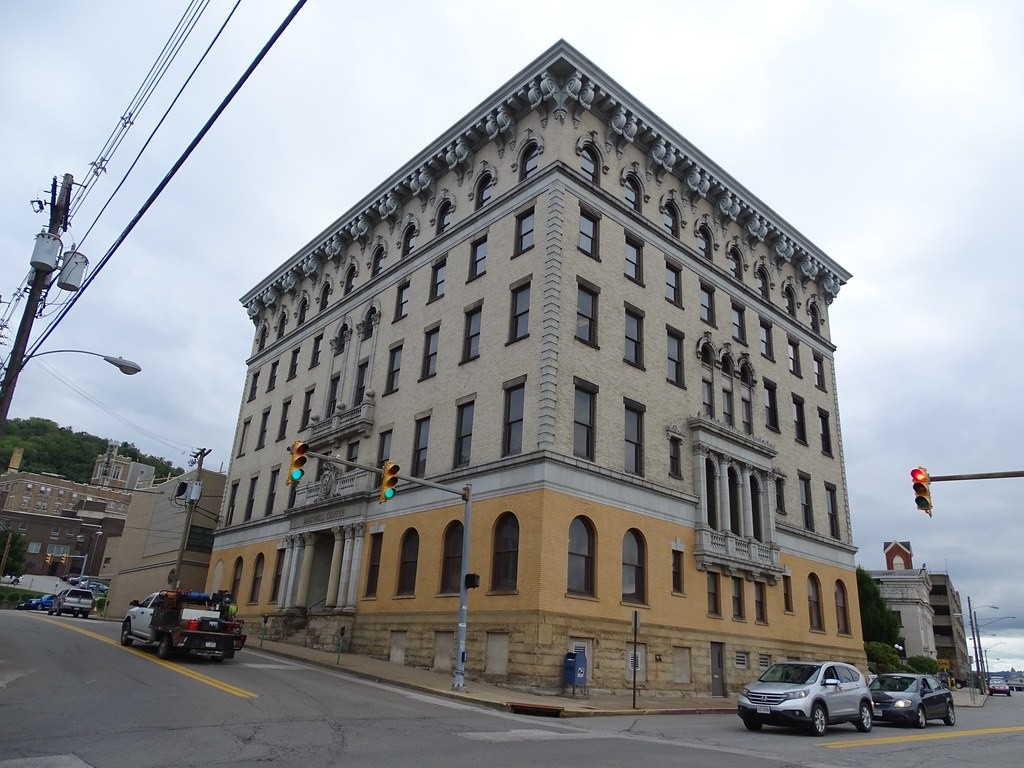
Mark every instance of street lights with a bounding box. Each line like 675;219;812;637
894;644;904;665
972;610;1016;692
0;350;141;438
967;595;1000;695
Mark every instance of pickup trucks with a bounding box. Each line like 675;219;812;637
120;590;248;664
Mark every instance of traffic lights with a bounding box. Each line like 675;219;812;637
285;441;309;489
45;553;54;565
379;461;401;506
910;467;933;512
464;573;481;590
61;554;69;565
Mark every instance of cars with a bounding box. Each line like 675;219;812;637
59;573;109;592
0;572;24;585
988;676;1011;697
17;601;27;610
935;671;967;689
870;673;956;729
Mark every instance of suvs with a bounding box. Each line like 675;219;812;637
52;587;95;619
737;660;875;737
26;594;56;611
